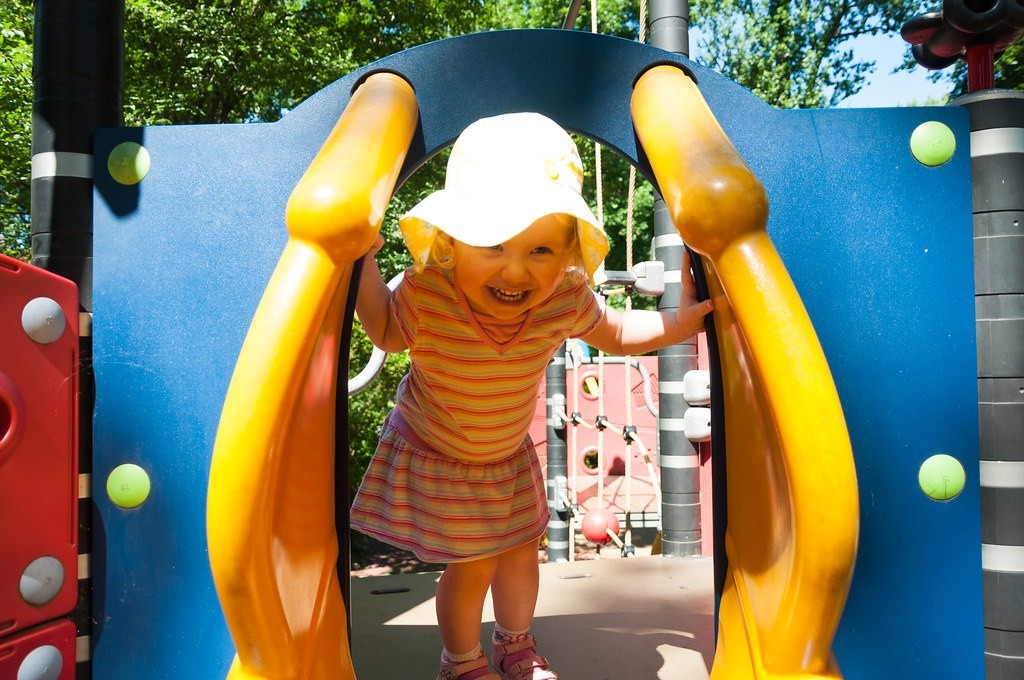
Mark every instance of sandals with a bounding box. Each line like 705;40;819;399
491;635;558;680
435;645;500;680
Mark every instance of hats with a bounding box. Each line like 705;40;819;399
401;111;610;290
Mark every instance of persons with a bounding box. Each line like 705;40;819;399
349;112;717;680
